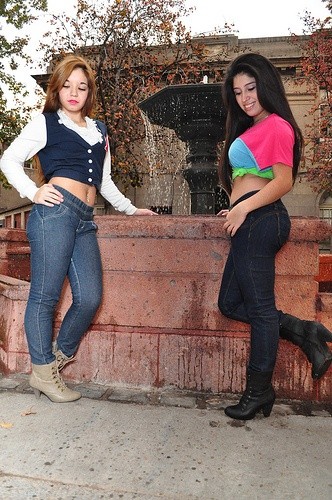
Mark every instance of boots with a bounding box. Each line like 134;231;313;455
225;367;274;420
280;313;332;379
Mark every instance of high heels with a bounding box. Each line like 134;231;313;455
50;341;73;373
29;363;82;403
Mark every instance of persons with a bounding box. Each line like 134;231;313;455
0;57;159;402
217;53;332;420
